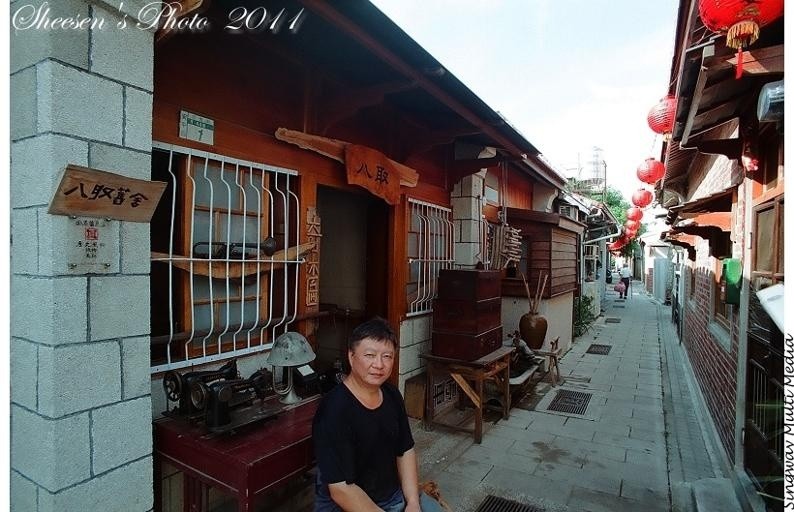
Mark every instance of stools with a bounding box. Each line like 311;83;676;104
531;336;562;389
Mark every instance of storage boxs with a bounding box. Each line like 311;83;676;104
431;269;503;362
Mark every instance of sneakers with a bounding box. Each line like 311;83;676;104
620;295;628;299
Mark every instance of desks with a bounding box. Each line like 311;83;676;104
418;346;516;444
152;392;324;512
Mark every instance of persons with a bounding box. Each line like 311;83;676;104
619;263;631;300
595;255;601;267
309;314;445;512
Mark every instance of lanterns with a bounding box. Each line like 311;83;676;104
607;208;643;251
636;157;666;186
695;0;784;80
632;188;654;209
646;94;678;143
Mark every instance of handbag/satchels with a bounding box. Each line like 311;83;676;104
613;282;626;292
